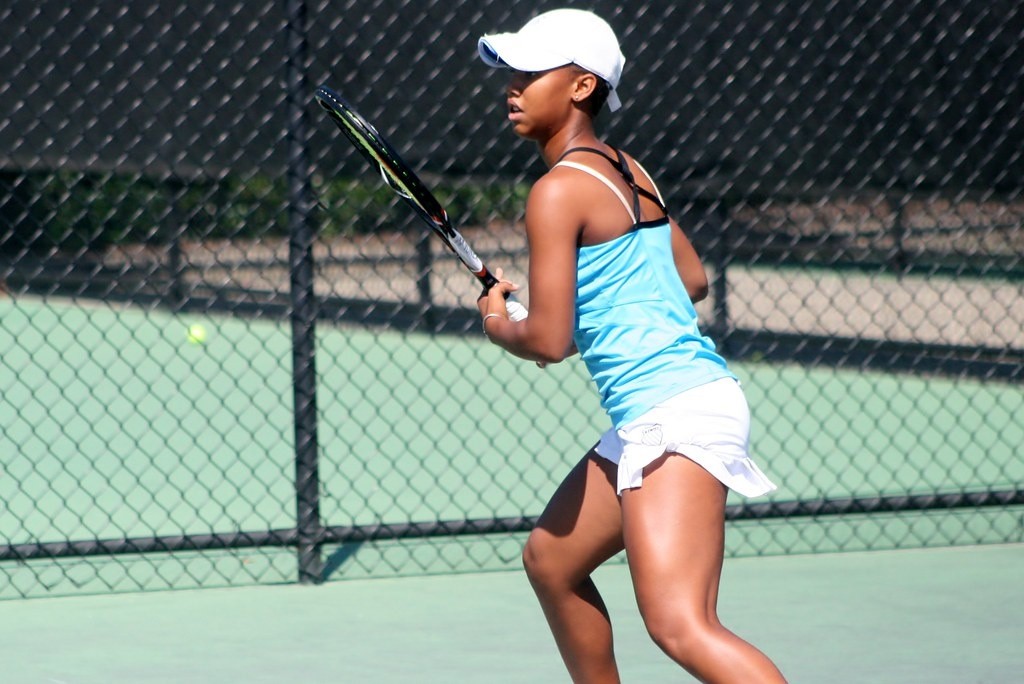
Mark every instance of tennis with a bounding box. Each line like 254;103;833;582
184;322;209;347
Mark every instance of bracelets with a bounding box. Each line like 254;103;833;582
482;313;505;337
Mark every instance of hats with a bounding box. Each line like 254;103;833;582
478;9;626;112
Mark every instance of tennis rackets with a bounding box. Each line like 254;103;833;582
313;83;530;322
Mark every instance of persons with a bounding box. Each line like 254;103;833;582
466;7;790;684
